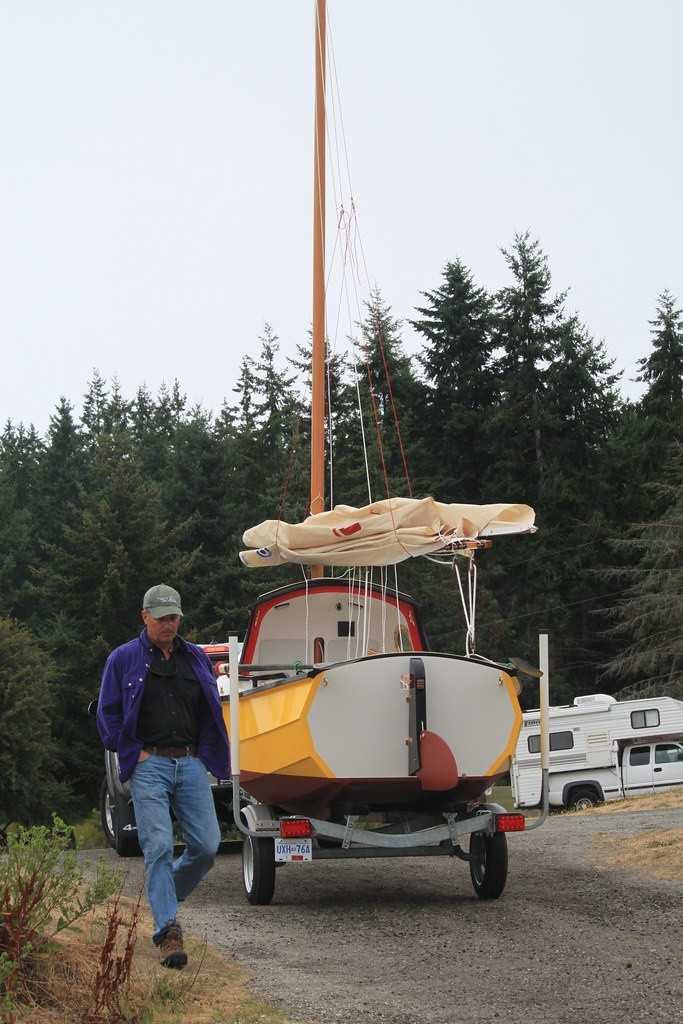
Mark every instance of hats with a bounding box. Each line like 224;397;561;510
142;583;185;619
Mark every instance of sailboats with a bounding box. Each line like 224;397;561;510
214;0;540;824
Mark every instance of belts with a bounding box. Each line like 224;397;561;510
144;744;198;757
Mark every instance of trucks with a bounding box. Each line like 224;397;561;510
87;629;554;906
508;694;683;814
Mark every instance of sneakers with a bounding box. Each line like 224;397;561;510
158;918;188;969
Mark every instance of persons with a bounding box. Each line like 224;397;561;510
96;583;233;966
